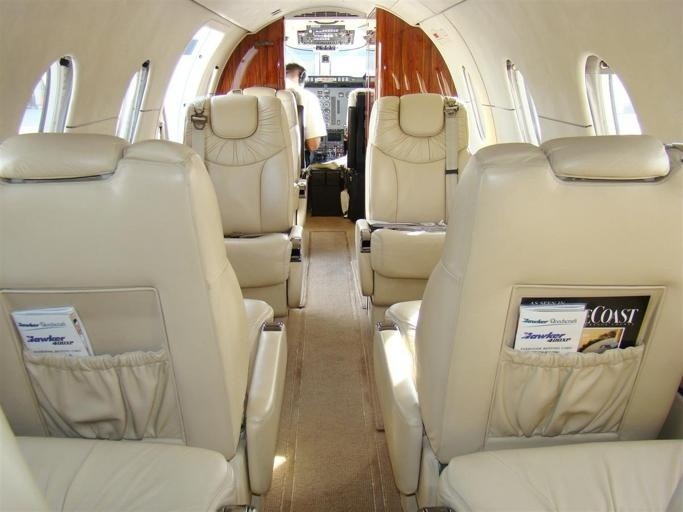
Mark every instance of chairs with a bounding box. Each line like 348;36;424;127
182;87;308;317
1;133;289;508
341;85;474;327
372;133;683;511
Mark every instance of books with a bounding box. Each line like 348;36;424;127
367;219;447;232
514;302;588;355
8;307;94;361
520;295;651;355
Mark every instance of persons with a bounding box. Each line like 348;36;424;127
283;62;329;169
